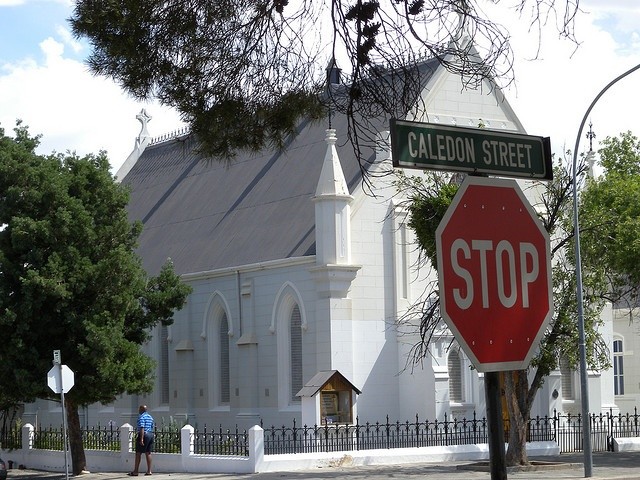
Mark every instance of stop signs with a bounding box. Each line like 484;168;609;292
434;176;554;372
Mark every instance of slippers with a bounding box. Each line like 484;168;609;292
145;473;152;475
129;472;138;476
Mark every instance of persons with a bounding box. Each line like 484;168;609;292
127;405;156;476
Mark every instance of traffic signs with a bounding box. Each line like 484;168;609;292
389;117;554;181
